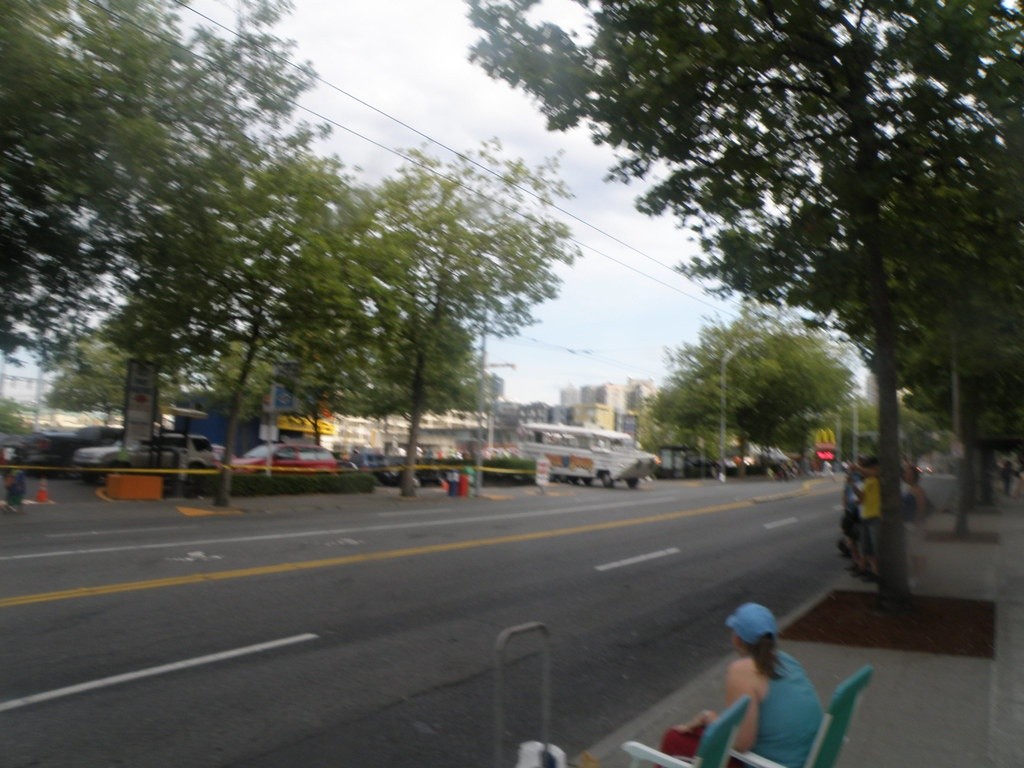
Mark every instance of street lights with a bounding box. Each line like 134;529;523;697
36;474;49;502
717;343;743;483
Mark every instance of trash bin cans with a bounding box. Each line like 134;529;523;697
938;475;959;514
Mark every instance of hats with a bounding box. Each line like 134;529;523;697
725;603;777;645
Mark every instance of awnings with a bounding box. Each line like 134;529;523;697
276;414;334;435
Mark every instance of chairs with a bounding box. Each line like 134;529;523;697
620;662;875;767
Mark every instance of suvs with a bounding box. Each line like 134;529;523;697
73;432;219;487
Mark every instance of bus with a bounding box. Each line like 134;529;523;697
518;422;660;489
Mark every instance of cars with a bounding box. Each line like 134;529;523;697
332;443;463;488
223;441;338;476
659;439;940;483
0;424;125;477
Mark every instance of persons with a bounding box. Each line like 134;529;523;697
689;602;824;768
0;447;28;512
1000;461;1019;494
837;455;925;583
